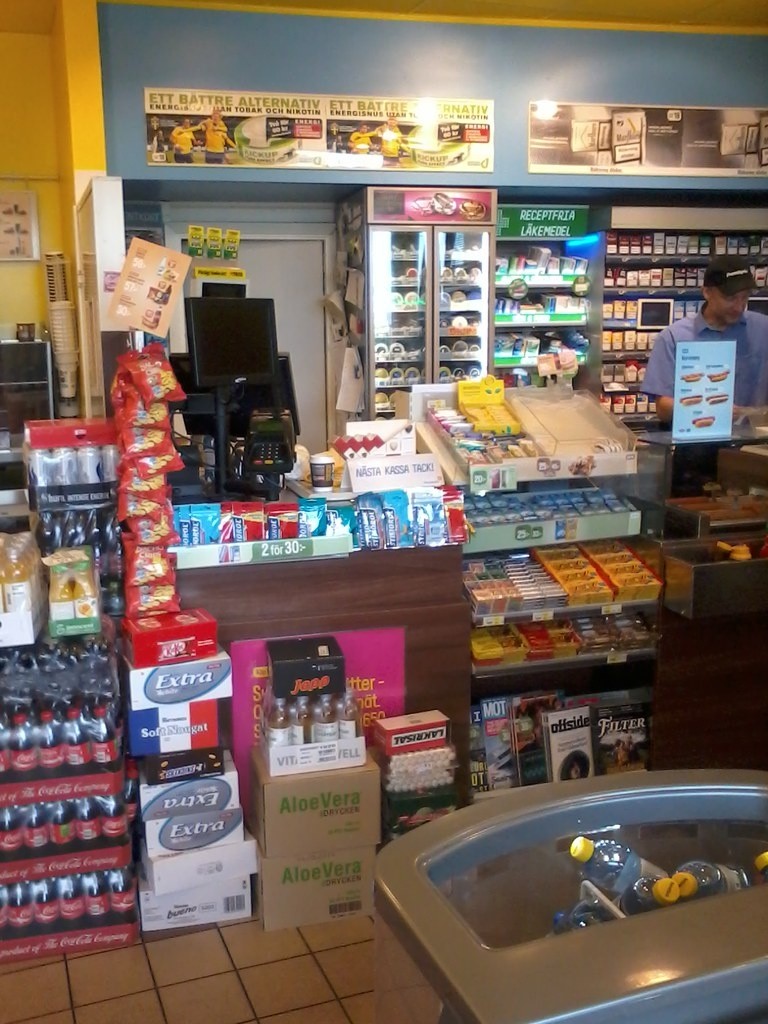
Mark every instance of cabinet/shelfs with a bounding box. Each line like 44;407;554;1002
411;379;662;794
566;202;767;424
494;203;590;387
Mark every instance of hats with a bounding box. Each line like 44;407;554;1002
703;255;758;293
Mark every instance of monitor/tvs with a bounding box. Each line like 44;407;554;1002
636;298;674;332
170;282;301;437
745;297;768;316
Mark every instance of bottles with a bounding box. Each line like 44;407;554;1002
755;851;768;882
611;875;681;915
0;635;109;673
0;704;125;778
0;867;140;940
0;794;128;859
554;911;587;934
569;836;668;897
124;760;139;826
35;509;123;618
265;688;358;747
1;532;101;618
0;676;114;711
553;912;566;924
672;860;751;901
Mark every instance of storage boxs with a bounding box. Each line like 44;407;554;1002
117;608;219;669
144;747;224;786
662;537;768;620
381;788;458;846
374;710;453;756
118;643;234;712
124;697;221;758
428;401;545;477
49;616;102;637
135;859;252;932
249;744;384;859
136;747;240;821
170;531;350;570
137;804;245;858
137;827;259;896
258;727;365;776
510;387;639;458
0;585;48;649
247;832;375;931
3;922;138;960
1;761;125;808
25;481;115;513
0;841;134;887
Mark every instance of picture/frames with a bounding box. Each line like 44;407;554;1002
1;188;40;261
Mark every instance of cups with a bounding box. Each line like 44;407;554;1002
309;454;335;492
16;323;35;342
43;251;78;398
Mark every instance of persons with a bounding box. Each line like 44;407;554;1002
640;251;768;429
328;115;403;166
158;108;236;165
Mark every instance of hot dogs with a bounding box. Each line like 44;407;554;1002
706;393;728;404
680;372;704;382
692;416;715;427
679;395;703;405
707;370;730;382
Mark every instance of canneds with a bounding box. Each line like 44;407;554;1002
28;443;120;486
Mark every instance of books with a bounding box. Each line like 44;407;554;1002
469;682;654;792
